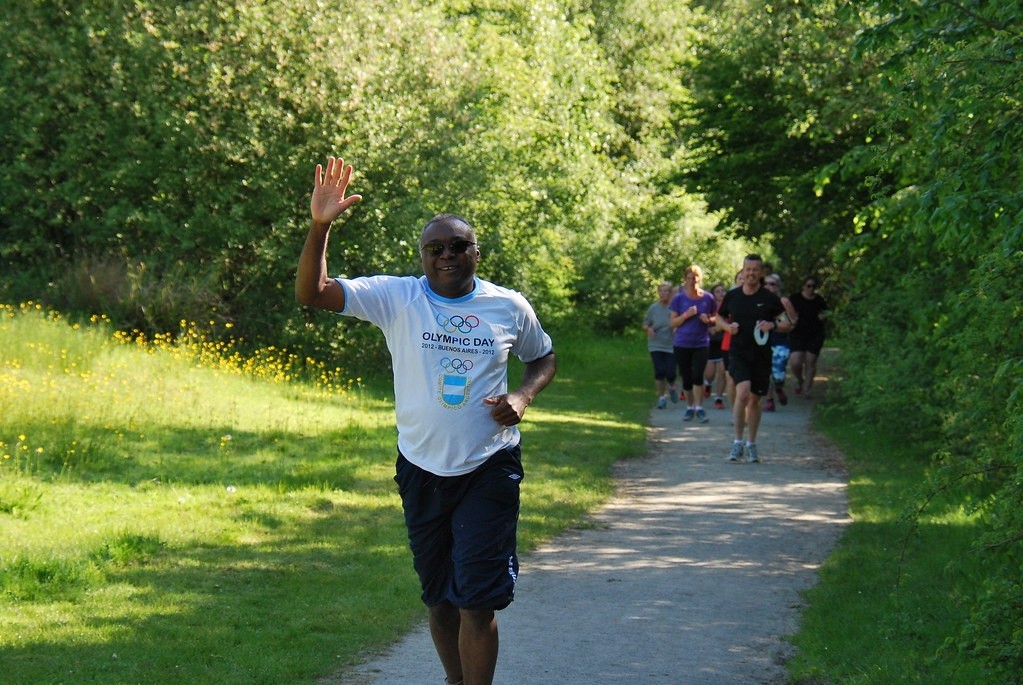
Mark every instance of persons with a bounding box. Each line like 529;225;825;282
759;275;798;413
718;254;789;464
704;284;730;406
669;263;716;425
721;269;746;425
293;156;558;682
786;277;827;397
643;284;679;408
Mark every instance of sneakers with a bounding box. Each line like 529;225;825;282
682;409;694;421
657;398;667;409
669;386;678;403
803;391;810;399
762;399;774;412
793;380;804;393
729;440;744;462
778;391;788;405
695;409;709;423
680;391;686;400
745;441;758;463
704;384;712;398
714;398;725;410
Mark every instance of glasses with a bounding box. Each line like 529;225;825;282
763;282;776;286
421;241;476;254
805;284;817;289
